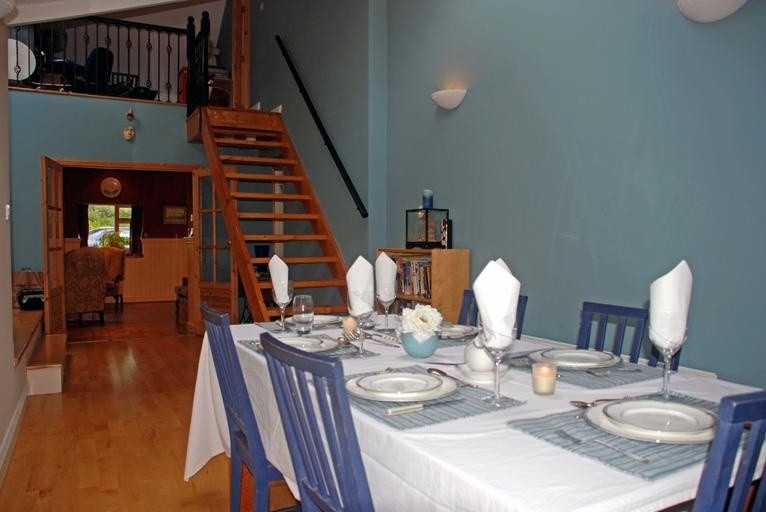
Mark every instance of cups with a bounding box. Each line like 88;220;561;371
292;295;314;335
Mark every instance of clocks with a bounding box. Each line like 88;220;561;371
100;177;122;198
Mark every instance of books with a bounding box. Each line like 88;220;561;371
389;297;430;317
389;255;433;300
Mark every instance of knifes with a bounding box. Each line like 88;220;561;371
383;397;466;416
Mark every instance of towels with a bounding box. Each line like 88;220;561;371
345;252;398;318
649;259;692;350
268;254;290;304
472;258;521;349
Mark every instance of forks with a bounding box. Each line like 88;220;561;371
555;428;649;463
344;331;382;345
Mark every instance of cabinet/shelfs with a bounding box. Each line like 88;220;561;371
376;247;470;322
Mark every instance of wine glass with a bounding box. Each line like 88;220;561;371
271;280;295;333
477;309;519;406
647;306;689;404
345;289;376;357
374;278;398;334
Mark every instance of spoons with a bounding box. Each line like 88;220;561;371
337;337;380;357
569;398;623;409
427;368;465;385
364;332;400;349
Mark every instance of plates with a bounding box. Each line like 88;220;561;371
279;336;323;348
283;314;343;328
527;346;623;370
437;324;479;338
356;371;442;395
271;336;339;353
602;398;719;438
541;348;614;365
458;363;510;381
584;398;718;445
344;371;458;402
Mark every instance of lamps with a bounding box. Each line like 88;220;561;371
677;0;747;23
429;90;466;110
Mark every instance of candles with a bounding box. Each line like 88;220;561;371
342;317;357;330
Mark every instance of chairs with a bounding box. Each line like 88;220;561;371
458;288;528;338
693;390;766;511
199;301;284;512
576;301;682;372
259;330;374;512
104;246;126;316
65;248;105;326
